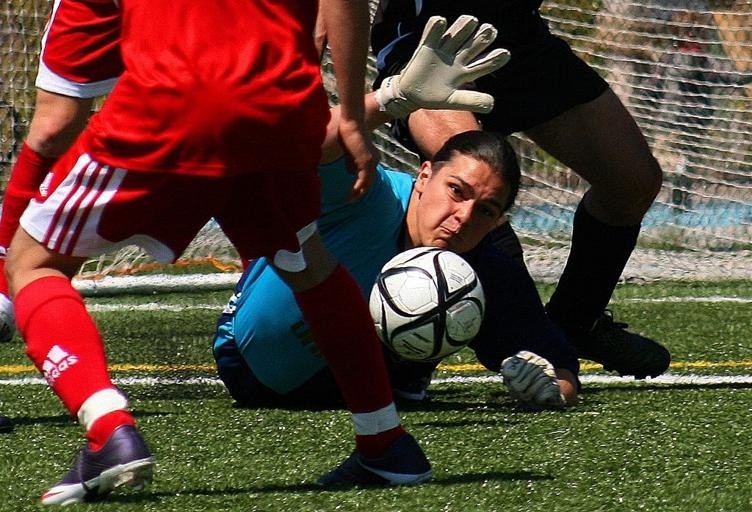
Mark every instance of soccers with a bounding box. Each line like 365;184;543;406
370;245;486;361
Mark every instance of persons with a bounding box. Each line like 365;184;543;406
213;19;577;412
371;1;671;380
0;0;432;507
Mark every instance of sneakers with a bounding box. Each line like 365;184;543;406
545;303;670;379
317;434;431;486
42;424;154;506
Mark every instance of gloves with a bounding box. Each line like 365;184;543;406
500;350;567;408
381;15;511;119
